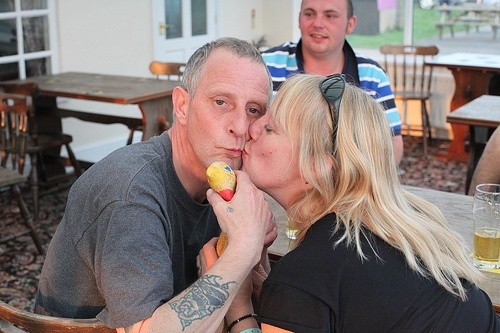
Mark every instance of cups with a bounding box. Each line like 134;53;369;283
285;211;300;240
474;183;500;270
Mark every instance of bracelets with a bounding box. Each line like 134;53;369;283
228;313;259;331
239;328;261;333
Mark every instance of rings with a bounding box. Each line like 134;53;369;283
196;266;201;271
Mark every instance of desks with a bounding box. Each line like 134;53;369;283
255;182;500;314
435;4;500;42
0;70;181;222
400;51;500;195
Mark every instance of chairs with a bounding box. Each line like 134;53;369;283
375;44;440;162
0;92;81;256
126;61;189;146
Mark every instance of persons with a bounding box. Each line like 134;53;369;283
32;36;273;333
261;0;403;167
196;72;500;333
467;123;500;197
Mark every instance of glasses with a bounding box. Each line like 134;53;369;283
320;73;346;155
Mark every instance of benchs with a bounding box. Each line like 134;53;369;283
435;16;499;30
